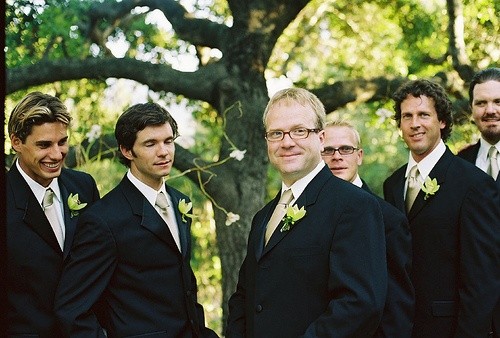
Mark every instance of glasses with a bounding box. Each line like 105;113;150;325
265;128;322;142
320;145;360;157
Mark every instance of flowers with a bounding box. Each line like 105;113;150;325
178;199;198;223
68;193;87;218
418;176;440;200
280;204;307;232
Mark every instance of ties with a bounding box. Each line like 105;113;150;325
263;188;294;247
484;146;500;180
404;164;421;215
41;188;64;251
155;192;182;254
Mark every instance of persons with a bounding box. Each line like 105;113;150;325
225;88;387;338
455;68;500;182
383;80;500;338
54;102;220;338
321;121;413;338
6;91;100;338
